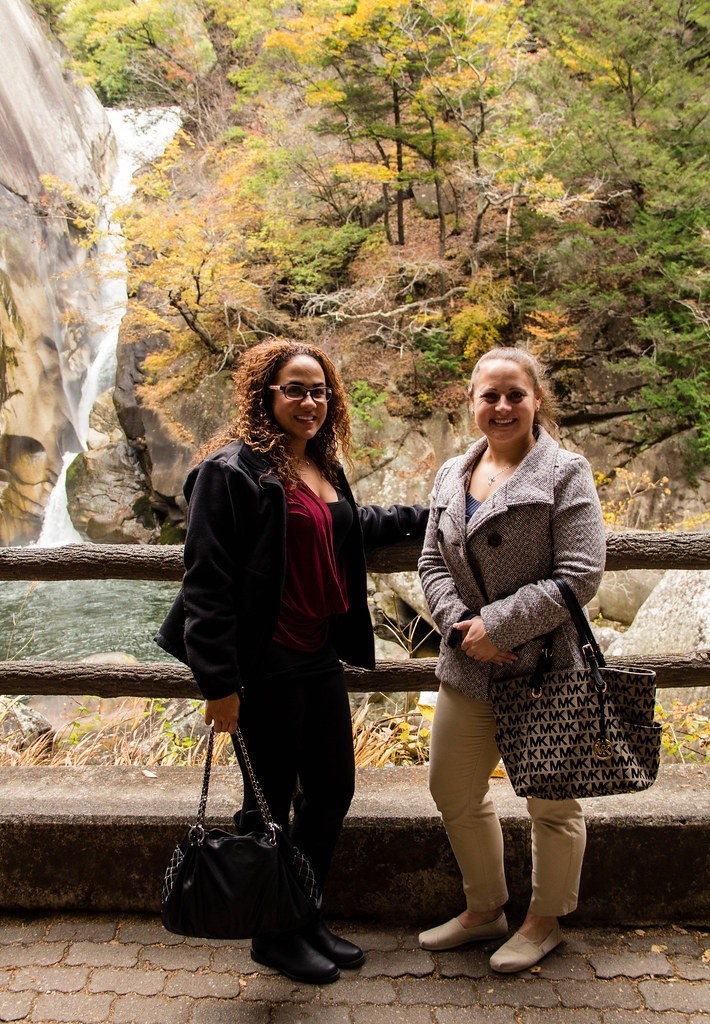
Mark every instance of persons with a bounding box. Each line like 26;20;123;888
417;344;607;974
152;338;428;985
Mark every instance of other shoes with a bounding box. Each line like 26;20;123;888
418;911;509;951
489;919;563;973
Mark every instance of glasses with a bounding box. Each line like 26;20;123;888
267;385;333;403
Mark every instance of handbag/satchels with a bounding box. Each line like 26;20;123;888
489;666;664;801
160;822;325;941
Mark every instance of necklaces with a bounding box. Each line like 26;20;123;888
484;451;517;487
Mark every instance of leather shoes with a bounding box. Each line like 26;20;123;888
250;925;366;985
